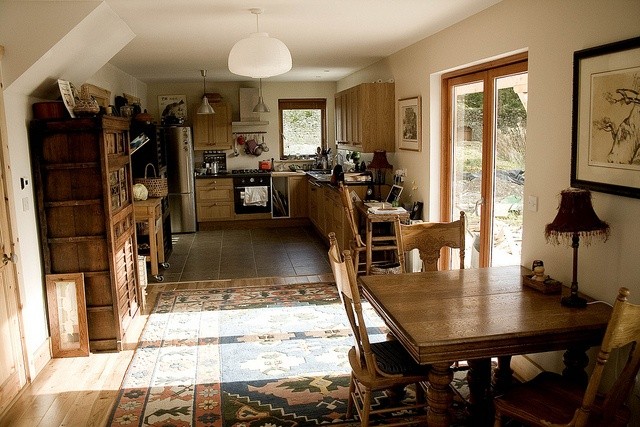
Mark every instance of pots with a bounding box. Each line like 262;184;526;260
200;159;220;175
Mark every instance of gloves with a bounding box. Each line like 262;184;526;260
247;139;258;154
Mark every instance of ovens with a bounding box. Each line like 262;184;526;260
234;186;271;214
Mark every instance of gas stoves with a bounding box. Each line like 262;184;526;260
232;169;271;186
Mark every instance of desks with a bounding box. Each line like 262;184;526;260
357;266;613;427
353;201;411;275
134;197;165;276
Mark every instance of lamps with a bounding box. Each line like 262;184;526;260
253;78;271;113
227;9;292;79
196;70;216;115
544;190;611;309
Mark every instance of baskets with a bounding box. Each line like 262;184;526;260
133;163;168;198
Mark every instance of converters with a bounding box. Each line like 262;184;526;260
395;170;399;177
398;169;403;176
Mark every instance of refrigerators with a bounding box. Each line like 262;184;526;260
161;126;197;235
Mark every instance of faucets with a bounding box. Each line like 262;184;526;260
335;153;343;163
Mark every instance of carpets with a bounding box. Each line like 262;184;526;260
106;283;538;425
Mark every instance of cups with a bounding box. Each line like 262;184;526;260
105;106;112;116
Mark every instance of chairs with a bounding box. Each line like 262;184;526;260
492;286;640;427
337;180;398;277
395;212;466;273
326;229;454;426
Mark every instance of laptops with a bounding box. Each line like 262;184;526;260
364;185;403;208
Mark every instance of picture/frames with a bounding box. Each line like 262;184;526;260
569;36;640;199
157;94;188;122
396;96;422;152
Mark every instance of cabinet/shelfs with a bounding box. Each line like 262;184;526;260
29;115;144;349
335;83;395;153
192;103;233;150
308;177;390;251
195;178;233;222
289;176;308;218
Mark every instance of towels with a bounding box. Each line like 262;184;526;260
243;186;268;208
